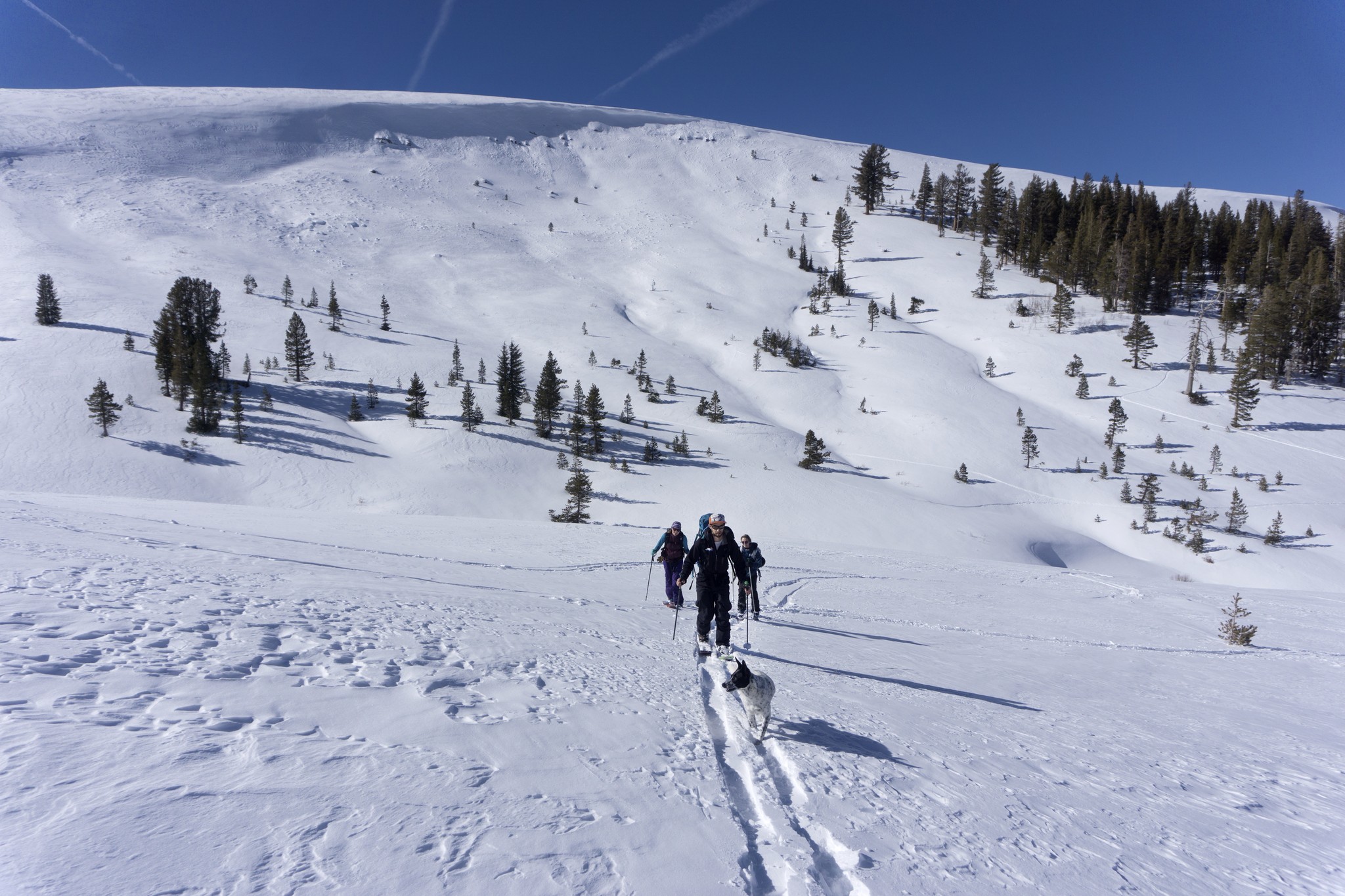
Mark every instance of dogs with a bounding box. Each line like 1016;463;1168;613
722;656;776;744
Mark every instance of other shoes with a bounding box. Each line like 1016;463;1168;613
738;610;744;614
753;612;759;617
669;599;674;602
675;603;682;608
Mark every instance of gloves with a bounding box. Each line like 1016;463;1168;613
747;561;753;567
650;549;656;555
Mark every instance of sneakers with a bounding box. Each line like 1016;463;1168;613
698;633;709;643
717;645;729;654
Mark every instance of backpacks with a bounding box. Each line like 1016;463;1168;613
698;513;713;539
740;545;761;562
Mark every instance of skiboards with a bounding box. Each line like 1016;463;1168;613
695;629;734;662
661;599;682;610
737;614;760;622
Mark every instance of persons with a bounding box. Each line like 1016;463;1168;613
650;514;766;624
676;514;752;656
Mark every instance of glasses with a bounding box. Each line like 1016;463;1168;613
741;540;749;544
673;528;681;532
710;524;725;529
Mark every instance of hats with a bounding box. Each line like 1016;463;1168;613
671;521;681;530
709;514;727;524
